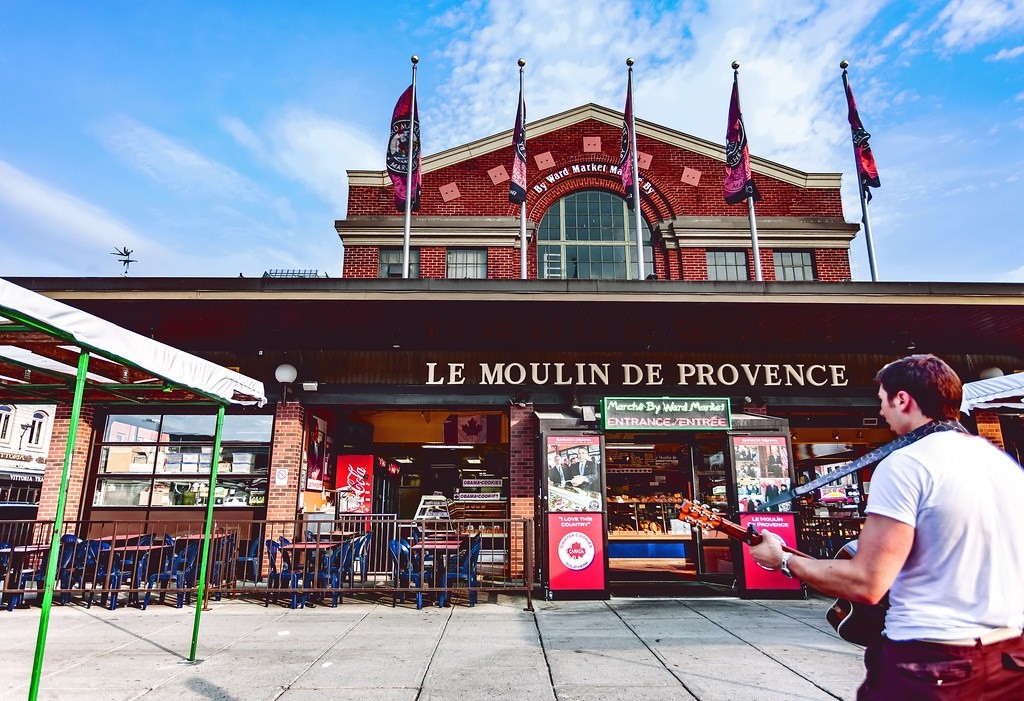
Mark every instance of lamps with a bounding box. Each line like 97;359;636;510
274;365;297;406
979;366;1004;381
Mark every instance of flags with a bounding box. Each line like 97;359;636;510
441;414;502;448
723;80;762;203
840;75;881;204
620;75;635;211
508;89;528;203
386;81;423;212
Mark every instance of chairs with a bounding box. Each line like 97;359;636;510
0;533;174;610
265;530;327;609
400;538;421;570
440;537;480;607
230;532;264;584
300;531;373;609
189;537;229;601
142;541;199;610
389;540;425;610
411;531;434;567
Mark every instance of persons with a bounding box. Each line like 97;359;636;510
734;444;759;477
548;446;603;493
766;446;783;479
750;354;1024;701
765;479;791;512
739;480;763;512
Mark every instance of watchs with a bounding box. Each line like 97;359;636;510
780;552;796;578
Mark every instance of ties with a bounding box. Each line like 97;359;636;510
558;467;566;485
581;464;584;475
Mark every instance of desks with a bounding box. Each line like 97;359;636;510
0;543;61;609
419;541;461;580
100;545;171;607
278;543;337;608
412;545;460;605
312;531;360;568
93;532;148;562
178;533;229;585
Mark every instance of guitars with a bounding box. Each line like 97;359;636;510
675;498;891;647
